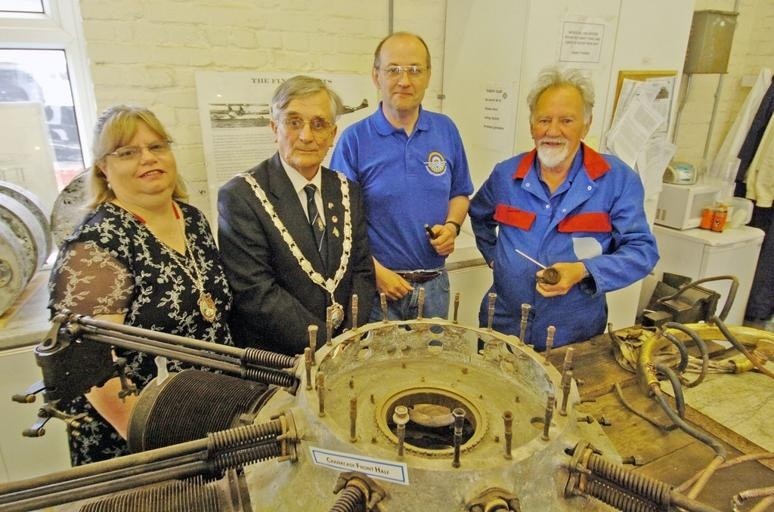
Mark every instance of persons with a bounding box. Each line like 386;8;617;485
326;28;474;323
216;73;378;364
46;102;236;470
466;64;664;352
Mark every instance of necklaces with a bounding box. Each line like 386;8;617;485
116;202;216;324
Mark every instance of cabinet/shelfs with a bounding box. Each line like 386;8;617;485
0;271;72;486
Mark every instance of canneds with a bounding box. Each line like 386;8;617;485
700;206;727;232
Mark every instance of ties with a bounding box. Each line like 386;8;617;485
303;184;327;273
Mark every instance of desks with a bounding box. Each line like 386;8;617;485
540;325;774;511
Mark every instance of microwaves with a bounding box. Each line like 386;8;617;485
654;176;736;229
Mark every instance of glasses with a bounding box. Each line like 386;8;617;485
104;136;173;160
381;65;428;80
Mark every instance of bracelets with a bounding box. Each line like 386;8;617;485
443;221;460;238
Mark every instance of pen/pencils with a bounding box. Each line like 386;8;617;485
424;223;436;240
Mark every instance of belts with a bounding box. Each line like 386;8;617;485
400;273;440;282
